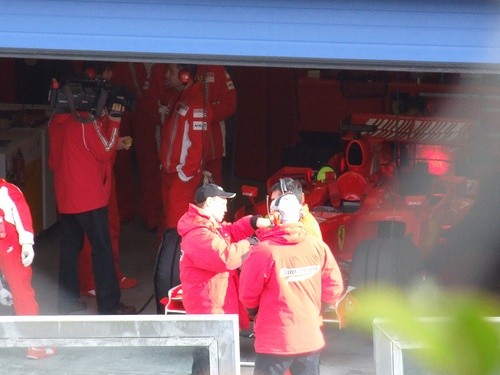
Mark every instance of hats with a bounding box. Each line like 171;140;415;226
194;184;236;203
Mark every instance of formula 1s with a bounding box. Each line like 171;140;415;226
152;123;490;346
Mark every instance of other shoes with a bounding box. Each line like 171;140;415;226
25;347;58;359
80;288;96;298
120;273;138;289
58;300;88;314
98;302;136;314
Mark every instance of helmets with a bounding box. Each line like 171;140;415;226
270;194;300;221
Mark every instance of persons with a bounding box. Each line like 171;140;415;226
84;61;237;248
177;183;272;375
0;178;57;359
49;103;136;314
239;191;344;375
271;177;322;241
77;108;138;295
153;63;213;315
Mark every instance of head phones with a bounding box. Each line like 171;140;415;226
268;191;292;227
178;64;194;87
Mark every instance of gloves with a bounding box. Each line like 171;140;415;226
21;243;35;267
